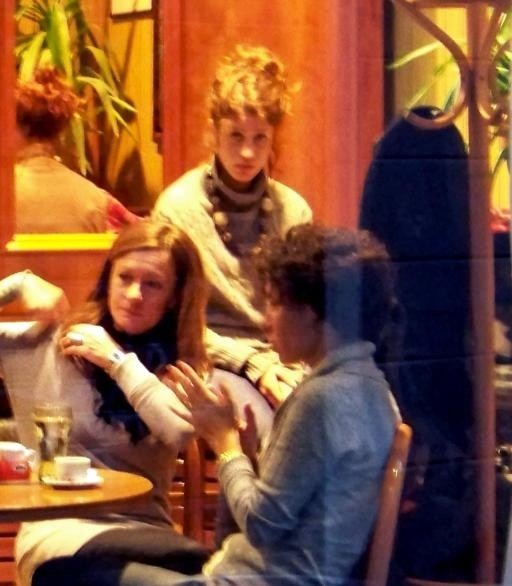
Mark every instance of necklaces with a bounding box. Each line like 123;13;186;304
200;165;275;260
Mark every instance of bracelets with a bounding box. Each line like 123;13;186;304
103;347;122;373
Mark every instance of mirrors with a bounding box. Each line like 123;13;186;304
0;0;180;251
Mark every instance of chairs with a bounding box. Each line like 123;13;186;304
179;422;416;586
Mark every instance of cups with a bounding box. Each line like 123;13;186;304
51;455;91;477
33;405;70;462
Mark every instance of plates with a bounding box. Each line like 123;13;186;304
42;474;104;489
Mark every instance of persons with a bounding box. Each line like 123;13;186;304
29;224;402;586
12;66;126;232
1;214;214;586
148;40;314;545
